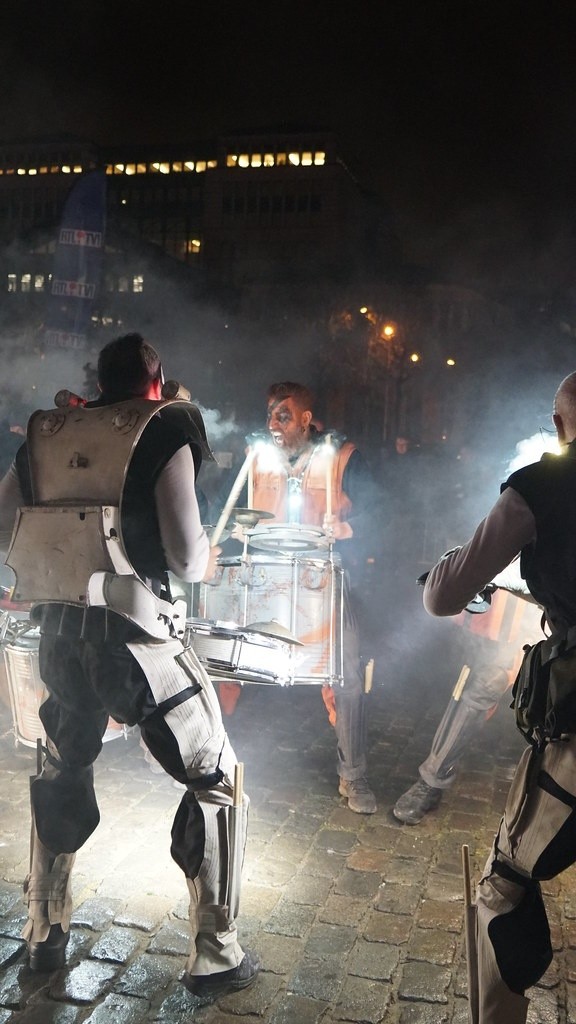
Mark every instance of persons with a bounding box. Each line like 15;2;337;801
142;382;390;814
0;334;262;996
371;372;576;1024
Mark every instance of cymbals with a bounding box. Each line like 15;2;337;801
220;506;276;527
200;526;232;547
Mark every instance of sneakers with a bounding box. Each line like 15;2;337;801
177;947;261;999
28;921;69;972
338;775;378;814
393;776;443;825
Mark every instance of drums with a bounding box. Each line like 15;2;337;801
245;522;327;552
195;551;342;689
2;625;135;750
